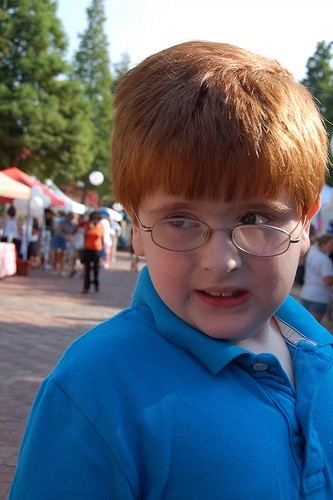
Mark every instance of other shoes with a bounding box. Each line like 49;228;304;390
96;287;99;291
81;290;88;293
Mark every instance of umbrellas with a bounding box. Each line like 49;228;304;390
0;166;131;271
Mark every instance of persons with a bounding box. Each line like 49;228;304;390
7;39;333;499
0;201;140;295
289;208;333;336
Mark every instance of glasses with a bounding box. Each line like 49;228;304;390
130;204;309;257
